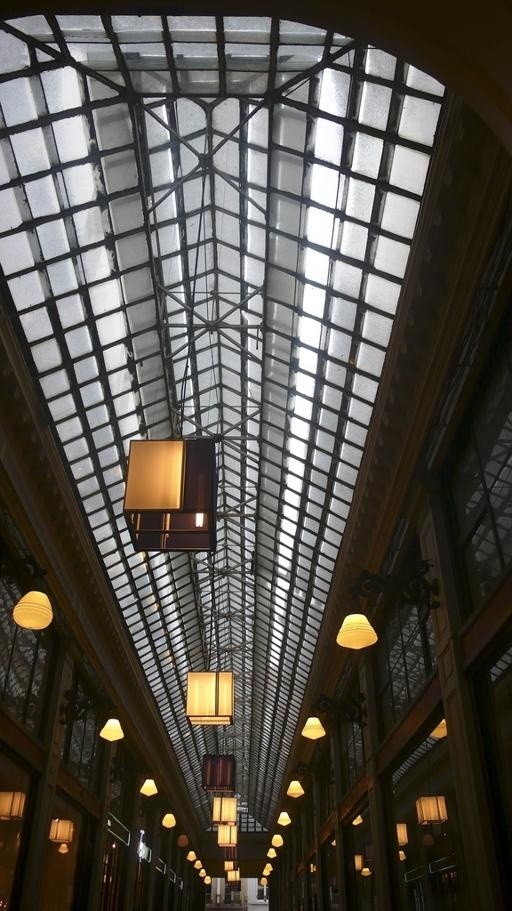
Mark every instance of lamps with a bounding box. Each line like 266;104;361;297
123;359;217;552
301;705;326;739
337;596;378;650
99;708;124;742
140;754;306;893
415;789;450;827
332;817;373;877
48;802;73;854
2;793;25;821
187;617;235;727
12;572;52;629
